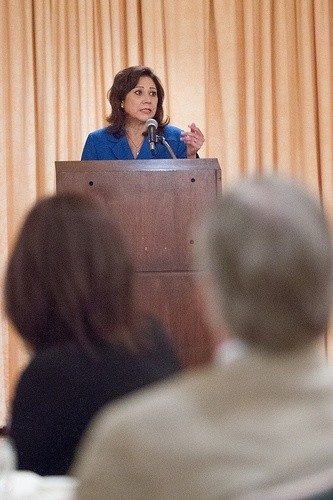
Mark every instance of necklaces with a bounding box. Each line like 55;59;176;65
126;130;144;155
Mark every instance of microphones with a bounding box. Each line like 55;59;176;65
145;119;158;150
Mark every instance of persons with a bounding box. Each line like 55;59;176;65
75;175;332;499
4;191;181;475
81;65;204;161
